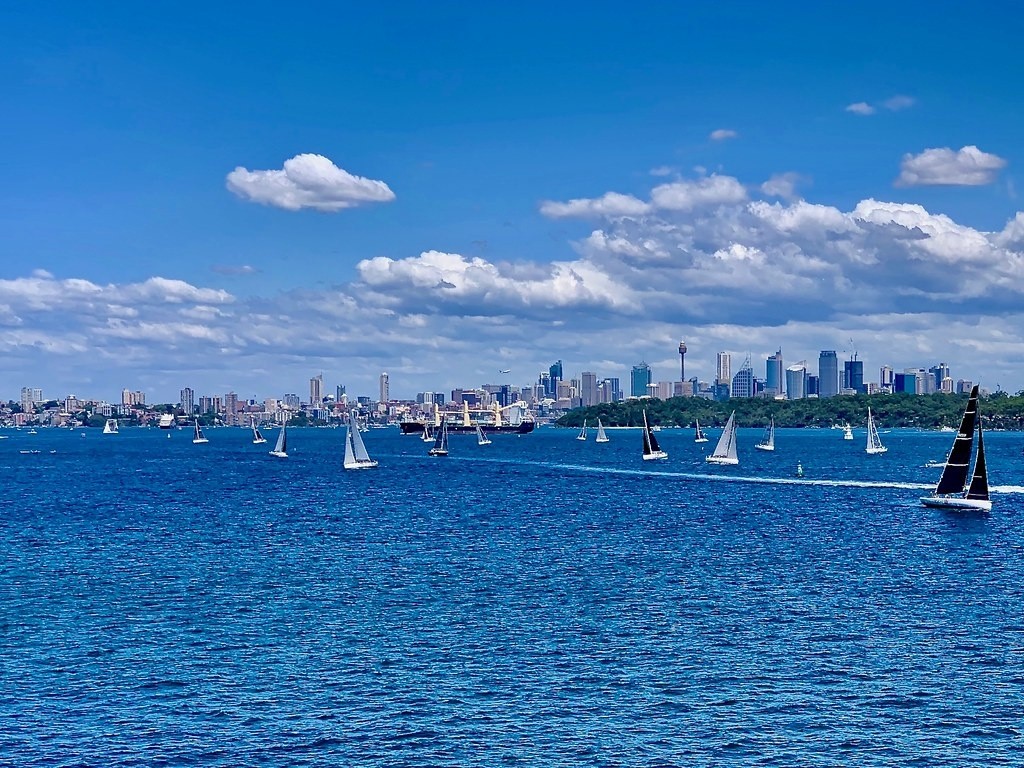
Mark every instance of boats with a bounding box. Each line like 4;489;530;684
102;418;119;434
400;399;534;437
160;413;176;429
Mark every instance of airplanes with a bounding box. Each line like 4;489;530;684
498;370;510;374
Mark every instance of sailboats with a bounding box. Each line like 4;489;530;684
252;419;268;444
865;406;888;455
640;408;669;461
576;418;588;441
694;418;709;443
596;419;609;443
343;409;379;470
426;411;449;457
919;384;992;513
754;415;775;451
192;418;210;443
476;422;492;446
269;423;289;458
705;410;739;466
